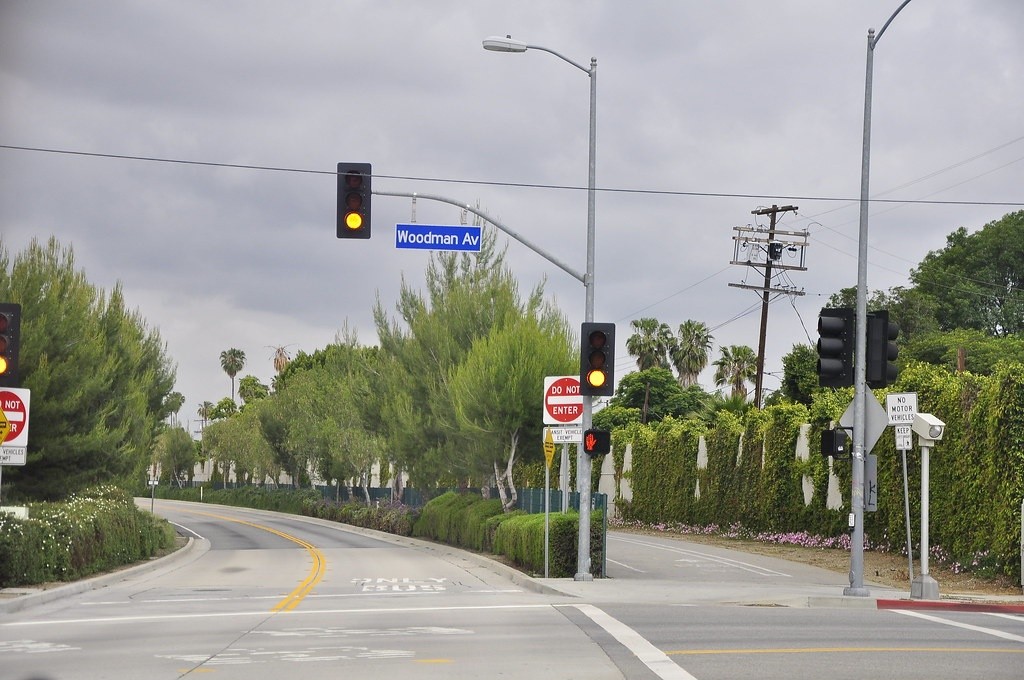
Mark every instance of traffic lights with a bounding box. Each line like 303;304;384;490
337;161;373;242
868;307;899;388
817;308;853;389
583;318;614;397
0;303;21;384
584;426;612;456
821;429;845;456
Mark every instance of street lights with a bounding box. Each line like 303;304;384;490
484;36;598;582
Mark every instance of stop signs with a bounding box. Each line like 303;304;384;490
0;387;31;446
540;371;584;423
